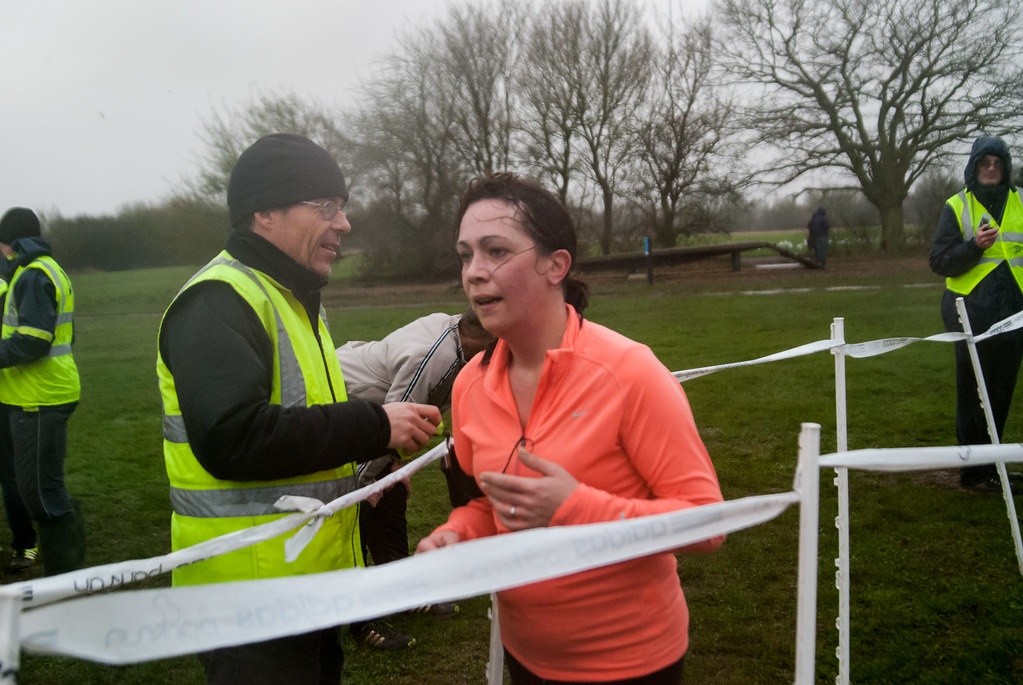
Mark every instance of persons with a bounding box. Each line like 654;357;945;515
156;133;443;685
808;208;828;265
414;173;727;685
1;208;85;578
336;307;493;646
930;136;1023;495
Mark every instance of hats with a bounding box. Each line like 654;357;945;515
0;206;42;245
225;132;349;226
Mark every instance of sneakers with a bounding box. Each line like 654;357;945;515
8;545;38;571
348;622;414;656
396;604;461;623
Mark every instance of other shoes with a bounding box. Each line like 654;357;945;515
959;470;1023;497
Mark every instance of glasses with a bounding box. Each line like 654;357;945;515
303;196;348;222
977;157;1004;170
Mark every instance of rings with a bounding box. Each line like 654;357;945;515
510;506;516;518
